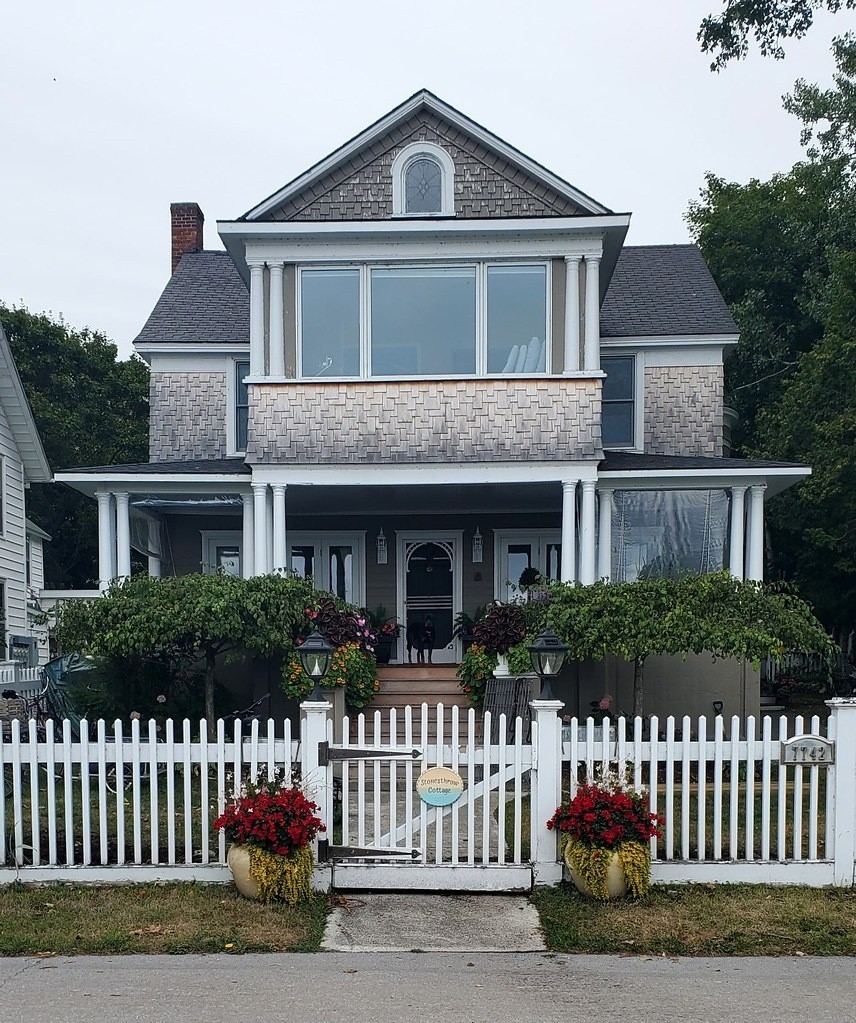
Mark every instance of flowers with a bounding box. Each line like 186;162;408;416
360;601;407;641
449;603;489;638
546;752;666;896
210;765;327;903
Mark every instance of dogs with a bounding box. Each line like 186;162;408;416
406;614;436;665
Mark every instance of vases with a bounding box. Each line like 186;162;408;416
374;638;394;666
461;635;480;652
564;842;631;897
229;844;265;899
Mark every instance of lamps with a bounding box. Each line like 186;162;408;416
470;526;484;562
296;625;336;703
375;525;389;566
523;623;572;701
